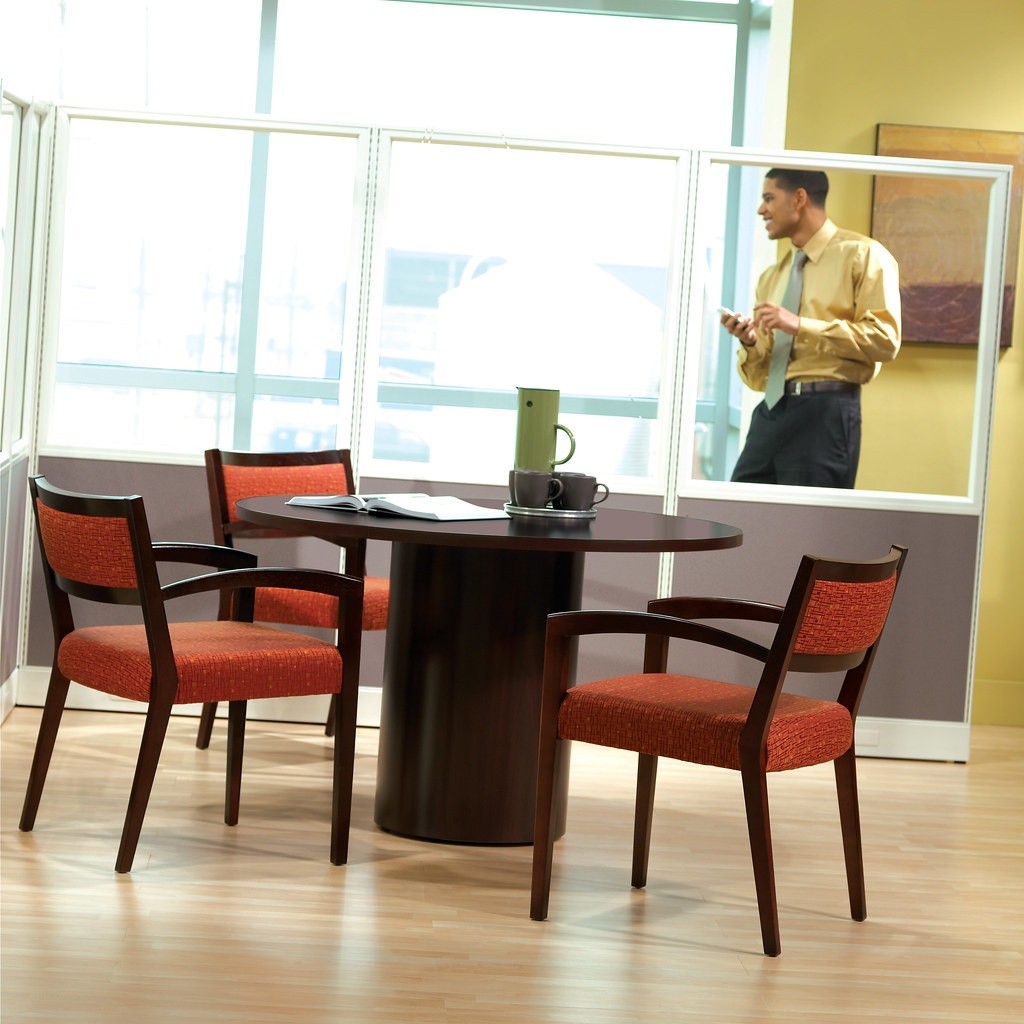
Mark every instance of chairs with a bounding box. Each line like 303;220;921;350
19;475;365;874
530;544;910;958
196;449;391;749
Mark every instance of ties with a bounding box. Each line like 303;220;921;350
764;249;809;413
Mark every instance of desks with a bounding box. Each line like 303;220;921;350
233;492;743;846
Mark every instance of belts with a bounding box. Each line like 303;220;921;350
781;378;859;398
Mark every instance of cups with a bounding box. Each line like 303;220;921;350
509;470;563;509
550;471;609;511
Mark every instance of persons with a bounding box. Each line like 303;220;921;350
721;169;904;489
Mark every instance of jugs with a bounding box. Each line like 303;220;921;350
514;386;576;473
